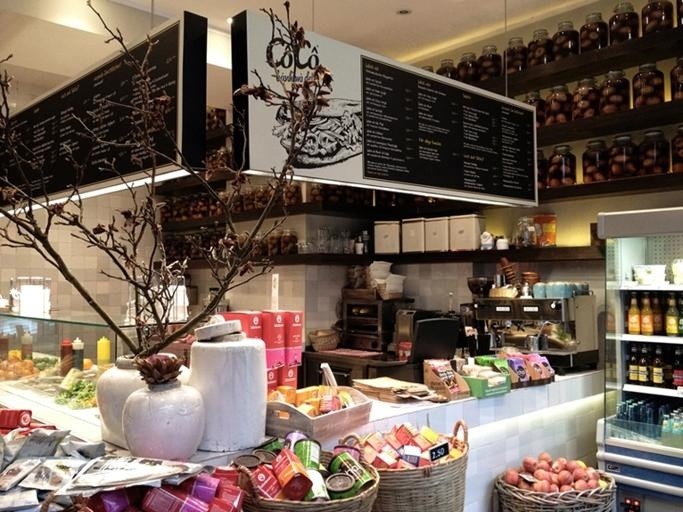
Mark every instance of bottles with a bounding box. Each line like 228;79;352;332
523;61;683;126
201;111;232;176
157;179;370;220
153;226;294;261
0;333;9;362
95;335;111;367
429;2;679;78
60;337;84;374
19;329;33;361
617;265;679;443
537;133;679;182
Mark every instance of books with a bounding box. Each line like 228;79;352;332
53;454;217;500
352;374;438;404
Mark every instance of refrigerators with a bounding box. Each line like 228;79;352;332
597;210;683;492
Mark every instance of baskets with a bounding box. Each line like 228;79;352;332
237;432;380;512
309;329;341;352
340;419;469;512
495;469;617;512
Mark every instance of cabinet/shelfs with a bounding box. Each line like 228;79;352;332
306;183;371;266
372;0;683;265
148;108;305;270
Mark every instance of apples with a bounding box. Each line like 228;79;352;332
503;453;601;494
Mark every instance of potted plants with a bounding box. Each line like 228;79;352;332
122;354;205;461
0;1;333;450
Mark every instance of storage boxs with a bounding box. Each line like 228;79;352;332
373;214;486;253
217;310;304;397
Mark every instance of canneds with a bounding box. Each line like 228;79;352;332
431;0;683;81
523;56;683;126
207;146;235;168
161;181;300;224
165;229;297;262
310;183;374;207
537;128;683;189
